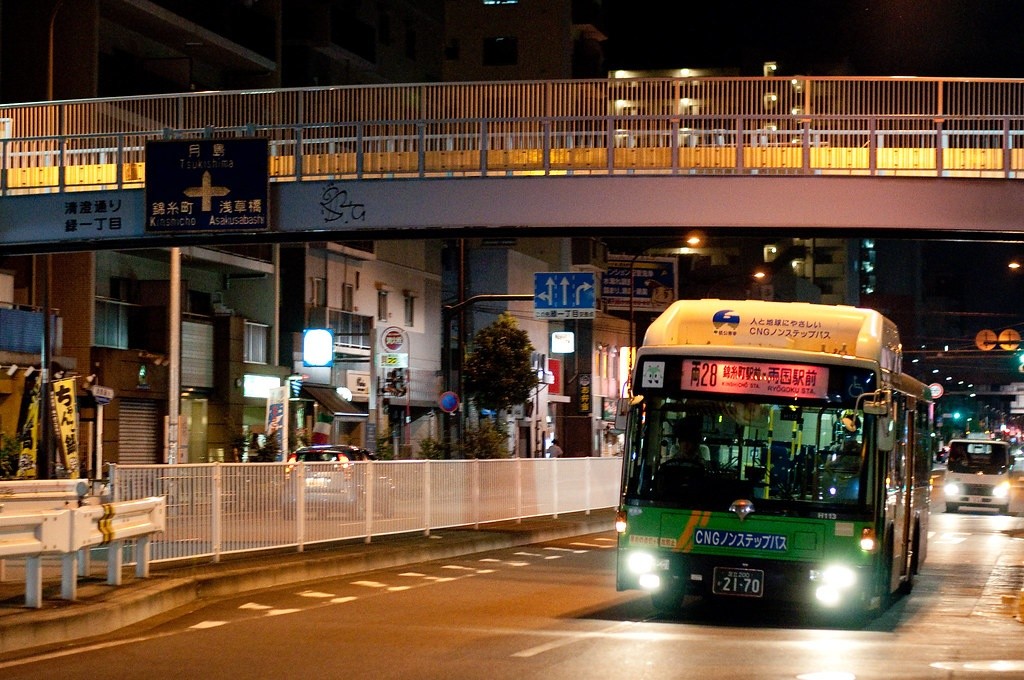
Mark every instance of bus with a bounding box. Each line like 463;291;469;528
615;298;934;629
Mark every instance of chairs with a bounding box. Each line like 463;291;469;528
762;446;827;493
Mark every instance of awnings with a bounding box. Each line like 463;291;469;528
302;382;370;422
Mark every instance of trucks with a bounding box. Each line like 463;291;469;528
944;439;1016;516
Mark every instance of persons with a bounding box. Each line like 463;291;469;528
546;438;563;458
652;416;720;511
823;437;861;490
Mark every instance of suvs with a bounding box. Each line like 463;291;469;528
280;444;395;520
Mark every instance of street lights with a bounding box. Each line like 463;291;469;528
1007;262;1024;273
628;238;700;371
706;271;765;297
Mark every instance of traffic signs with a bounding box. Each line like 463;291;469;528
91;385;114;400
